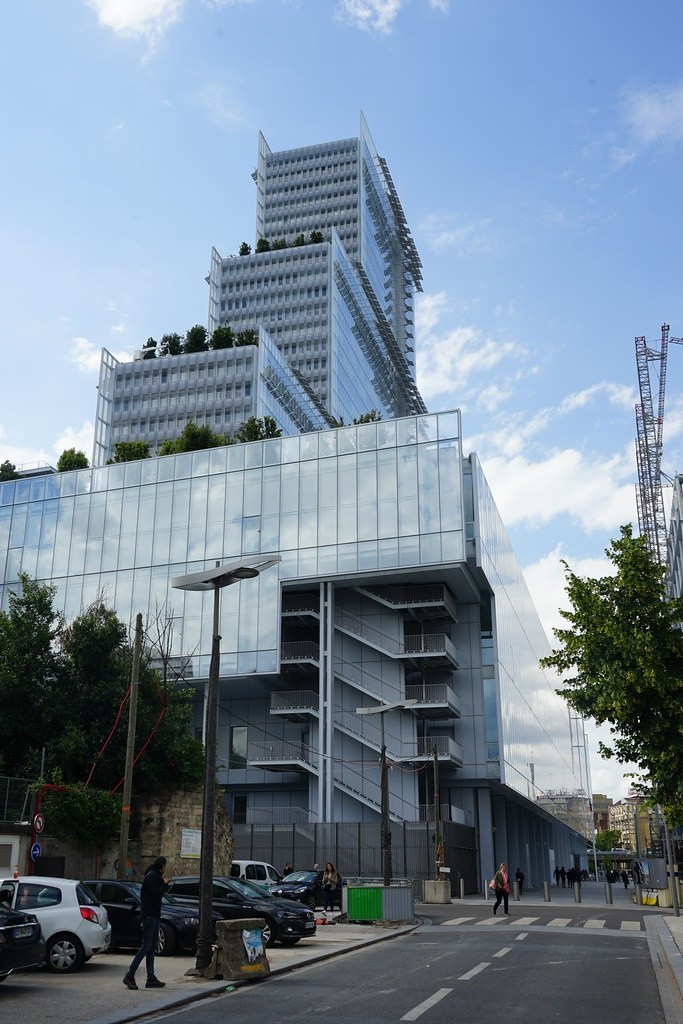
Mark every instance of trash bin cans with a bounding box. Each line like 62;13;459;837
598;871;606;882
341;877;415;923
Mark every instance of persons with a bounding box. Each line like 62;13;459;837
605;870;621;884
553;866;581;888
283;863;294;876
580;869;589;881
314;864;320;870
621;870;629;888
322;863;338;916
492;863;510;916
515;868;525;895
0;890;11;910
123;857;170;989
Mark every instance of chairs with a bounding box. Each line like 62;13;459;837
0;889;9;902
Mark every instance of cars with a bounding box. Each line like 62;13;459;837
163;876;317;948
0;904;47;983
267;870;343;912
0;876;112;972
38;879;223;957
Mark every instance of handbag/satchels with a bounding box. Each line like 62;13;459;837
489;871;500;889
324;884;332;890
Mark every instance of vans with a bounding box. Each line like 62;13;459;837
231;860;281;896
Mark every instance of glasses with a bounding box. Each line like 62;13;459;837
328;866;330;867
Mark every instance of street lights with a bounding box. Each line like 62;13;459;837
356;698;417;887
171;554;283;976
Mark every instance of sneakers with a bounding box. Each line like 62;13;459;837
146;978;166;988
123;974;139;989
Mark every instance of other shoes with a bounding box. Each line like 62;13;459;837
331;912;334;917
492;907;496;915
322;911;327;916
504;912;510;916
